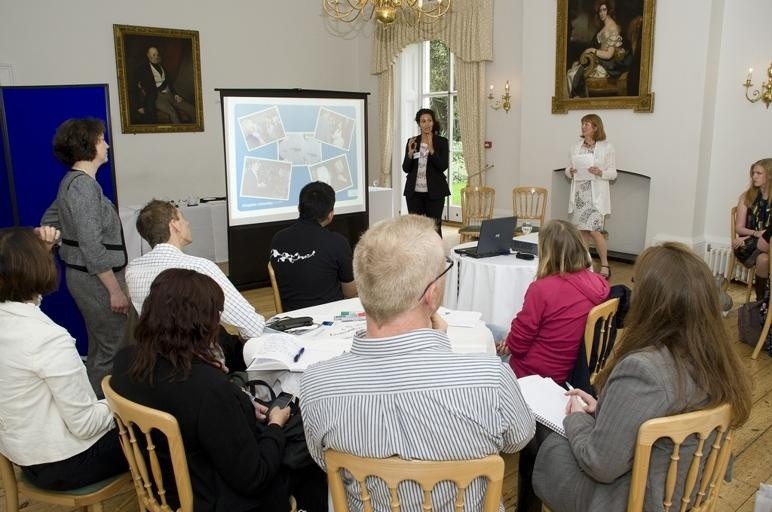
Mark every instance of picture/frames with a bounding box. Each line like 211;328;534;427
551;1;657;114
111;24;206;134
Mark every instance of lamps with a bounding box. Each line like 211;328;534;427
488;81;512;114
741;61;772;110
323;0;450;33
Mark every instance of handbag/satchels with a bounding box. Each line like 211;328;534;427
227;375;317;470
739;299;767;351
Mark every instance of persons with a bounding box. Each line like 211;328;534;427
564;113;619;278
139;47;196;122
108;266;329;512
0;224;140;492
39;116;133;401
267;180;359;314
756;224;772;358
519;240;752;512
239;110;354;197
730;158;772;310
568;1;628;101
496;218;611;382
294;214;537;512
118;200;270;376
401;108;451;240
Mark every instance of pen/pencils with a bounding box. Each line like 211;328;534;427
294;347;304;361
566;381;588;408
330;327;354;336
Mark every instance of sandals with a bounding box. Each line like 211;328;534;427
601;266;611;281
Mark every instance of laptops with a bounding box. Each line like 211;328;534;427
454;216;515;258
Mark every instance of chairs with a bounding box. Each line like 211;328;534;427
627;403;732;512
0;374;196;511
723;208;756;306
458;186;548;246
585;297;620;384
322;449;505;511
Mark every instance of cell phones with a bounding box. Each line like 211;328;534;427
265;391;294;415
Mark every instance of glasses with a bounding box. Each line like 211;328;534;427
418;255;453;301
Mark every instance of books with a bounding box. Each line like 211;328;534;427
441;308;484;329
241;335;352;372
513;373;587;438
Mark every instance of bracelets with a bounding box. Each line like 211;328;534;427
752;230;759;238
